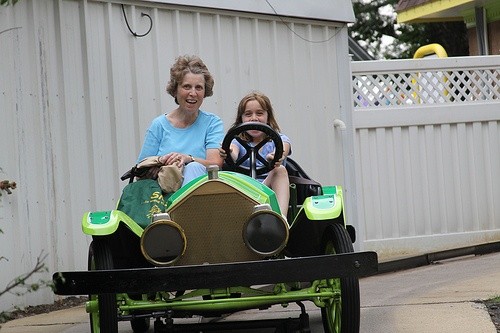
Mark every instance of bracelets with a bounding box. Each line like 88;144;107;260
185;155;194;165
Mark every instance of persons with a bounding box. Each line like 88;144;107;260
136;56;224;200
218;93;292;225
